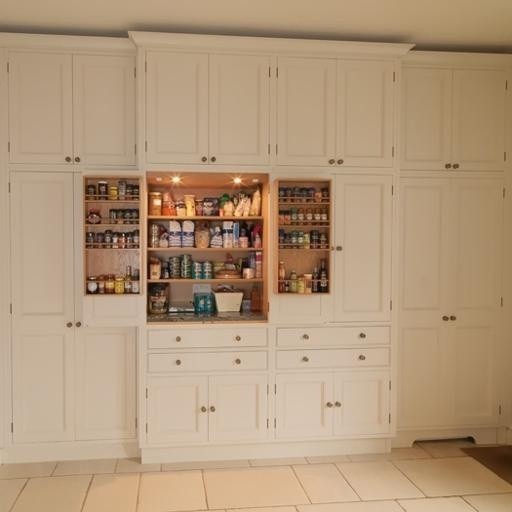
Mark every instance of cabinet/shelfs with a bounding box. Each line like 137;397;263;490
392;50;511;451
129;30;417;463
1;30;139;464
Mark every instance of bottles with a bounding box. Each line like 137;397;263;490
84;174;140;249
278;257;328;293
124;265;139;293
278;187;330;248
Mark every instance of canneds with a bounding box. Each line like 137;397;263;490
148;192;186;216
168;253;212;279
109;186;118;200
195;292;215;313
86;274;125;294
